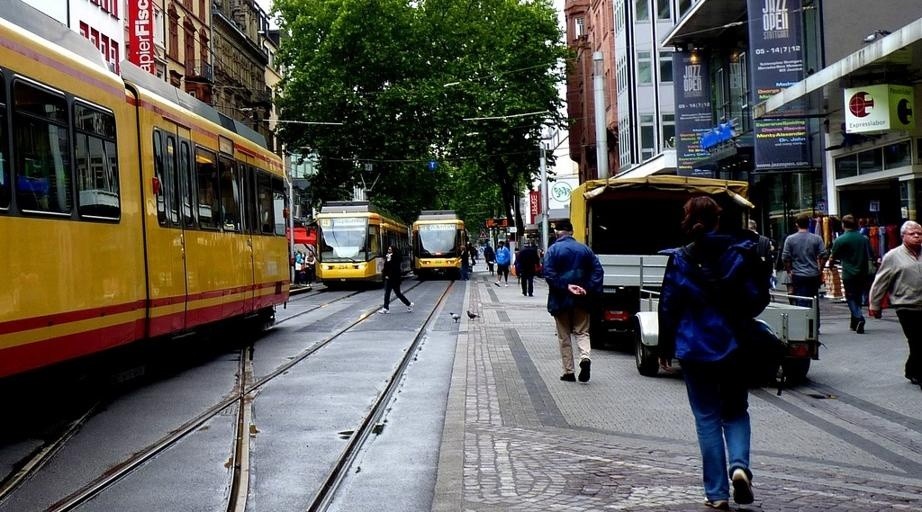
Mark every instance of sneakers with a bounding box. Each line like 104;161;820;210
495;281;501;287
560;373;576;382
856;320;865;334
732;468;755;505
578;358;591;382
705;496;729;510
505;283;509;287
378;308;390;314
407;302;414;313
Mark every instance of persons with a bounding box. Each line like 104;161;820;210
377;245;414;314
542;219;603;382
460;240;540;296
778;212;922;390
294;248;316;286
658;196;771;510
748;219;771;251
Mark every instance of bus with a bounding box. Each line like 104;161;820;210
0;0;295;391
314;197;412;290
409;208;470;278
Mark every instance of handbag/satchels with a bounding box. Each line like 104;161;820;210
739;317;783;388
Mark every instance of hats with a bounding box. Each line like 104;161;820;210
556;221;573;232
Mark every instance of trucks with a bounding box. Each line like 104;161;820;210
560;174;756;352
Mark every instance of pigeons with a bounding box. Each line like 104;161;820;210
449;312;461;323
466;311;480;321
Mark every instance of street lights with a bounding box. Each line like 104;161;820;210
538;141;550;254
